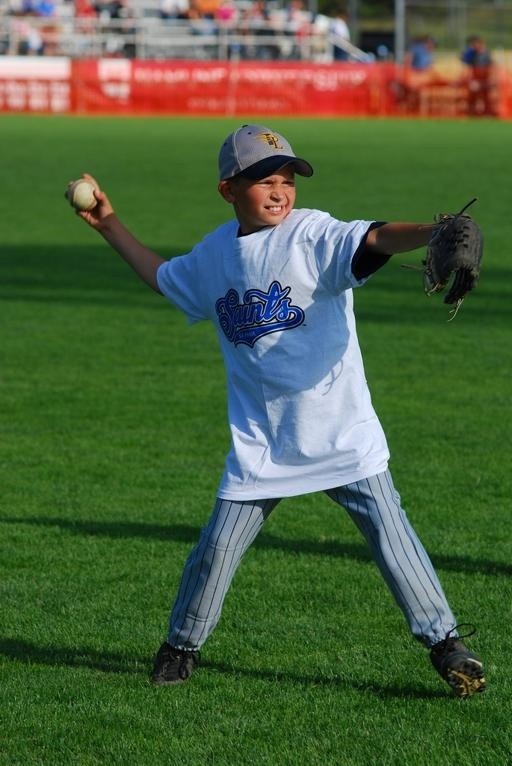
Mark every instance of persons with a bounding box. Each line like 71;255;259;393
463;36;497;117
0;0;351;63
409;34;434;74
60;121;487;701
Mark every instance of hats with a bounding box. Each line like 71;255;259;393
218;125;313;181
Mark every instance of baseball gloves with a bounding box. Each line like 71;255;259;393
400;196;484;322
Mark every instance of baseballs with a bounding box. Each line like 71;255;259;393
67;179;98;212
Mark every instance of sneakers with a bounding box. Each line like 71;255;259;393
431;624;486;698
152;641;201;683
